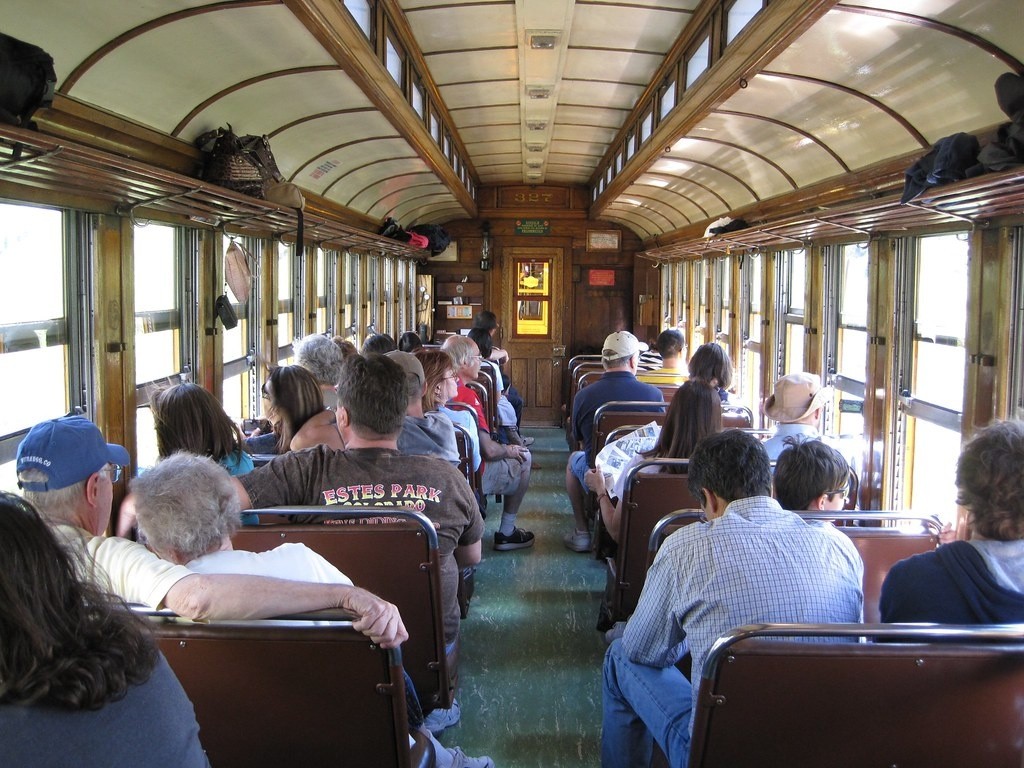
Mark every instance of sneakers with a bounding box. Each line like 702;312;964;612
520;435;535;445
564;528;592;552
445;746;496;768
493;526;535;550
423;698;462;735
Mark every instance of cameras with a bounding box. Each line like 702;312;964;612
243;419;271;435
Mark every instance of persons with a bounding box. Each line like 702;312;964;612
16;418;409;648
636;328;689;383
689;344;751;423
773;435;851;522
0;492;211;768
597;428;864;768
562;330;665;551
235;309;535;549
131;451;494;768
873;419;1024;644
150;383;259;524
118;353;485;655
584;380;721;545
760;372;854;474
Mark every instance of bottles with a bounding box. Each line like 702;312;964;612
419;325;427;343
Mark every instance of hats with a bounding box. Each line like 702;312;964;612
16;415;130;492
601;331;649;361
763;372;833;423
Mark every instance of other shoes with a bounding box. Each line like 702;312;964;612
530;461;541;469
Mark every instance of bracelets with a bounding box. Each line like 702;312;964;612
597;492;606;502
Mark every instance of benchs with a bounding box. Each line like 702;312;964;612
560;355;1024;768
83;344;504;768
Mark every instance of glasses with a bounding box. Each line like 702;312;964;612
467;355;483;361
261;382;272;400
99;463;121;483
681;344;688;351
823;483;850;498
698;495;708;523
442;371;456;382
